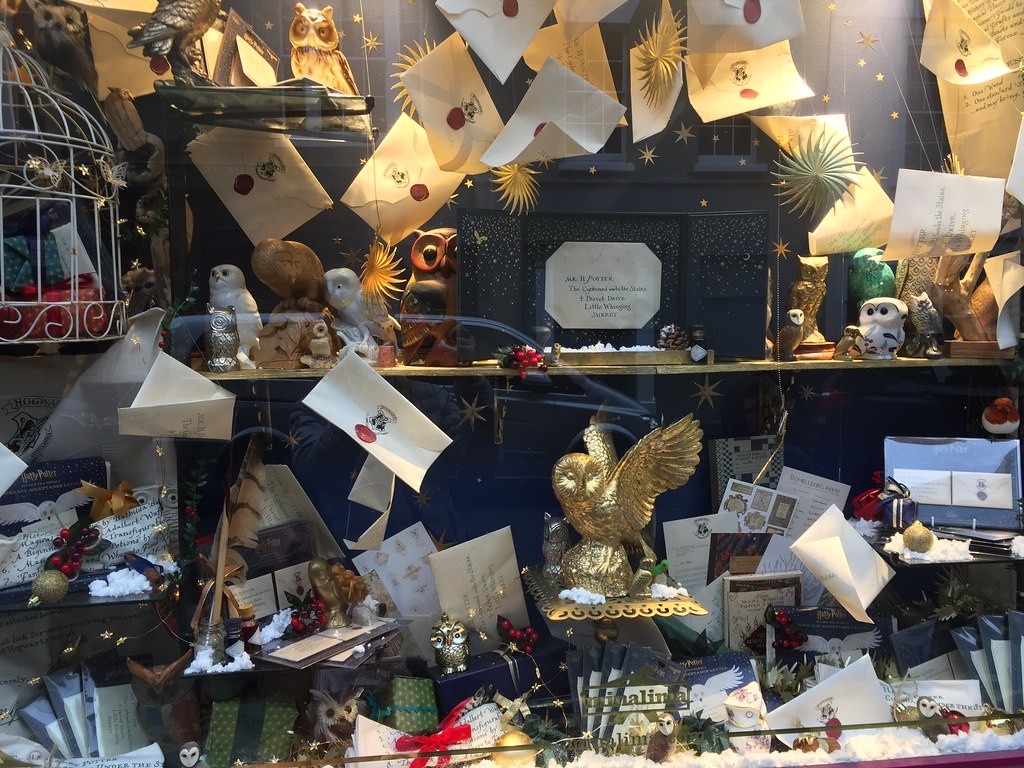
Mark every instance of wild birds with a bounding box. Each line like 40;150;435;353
982;398;1020;443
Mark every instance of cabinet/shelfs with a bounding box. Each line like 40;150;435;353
1;338;175;613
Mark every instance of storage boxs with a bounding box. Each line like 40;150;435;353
202;634;576;768
